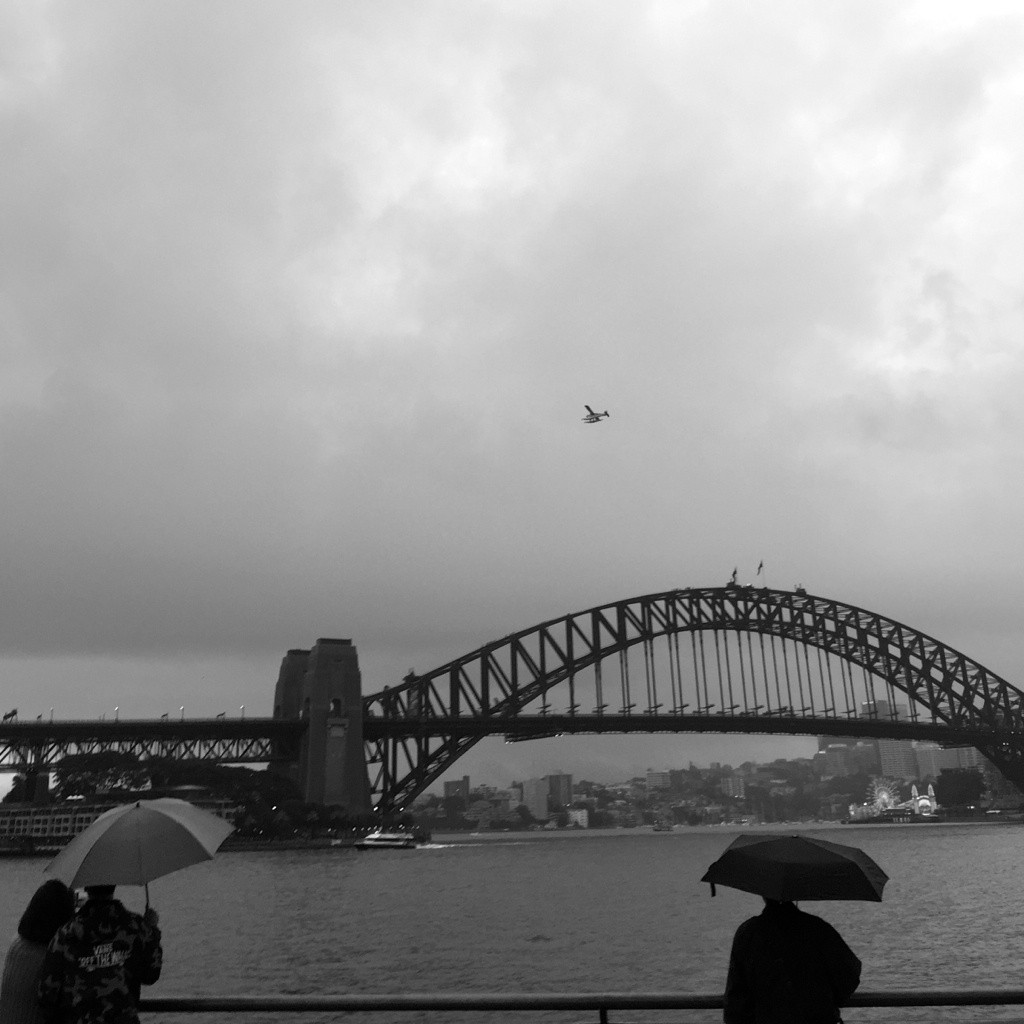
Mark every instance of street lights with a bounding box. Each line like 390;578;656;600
240;705;244;719
115;706;119;720
180;706;184;719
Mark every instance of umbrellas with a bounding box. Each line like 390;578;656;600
700;834;890;908
42;798;236;910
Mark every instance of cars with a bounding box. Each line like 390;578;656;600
986;808;1000;814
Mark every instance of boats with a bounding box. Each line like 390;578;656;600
652;825;673;831
353;830;416;850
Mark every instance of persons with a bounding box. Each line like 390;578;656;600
722;896;862;1024
0;878;163;1024
